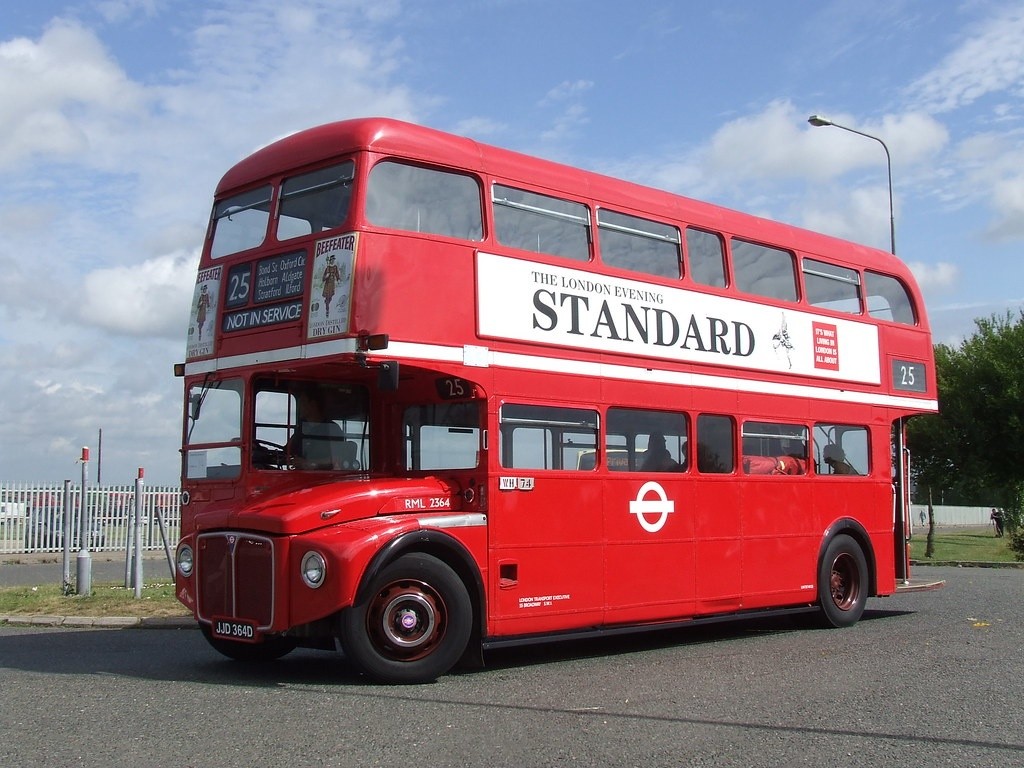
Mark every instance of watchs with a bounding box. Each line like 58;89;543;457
311;463;316;467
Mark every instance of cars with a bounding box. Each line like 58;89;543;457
24;505;106;553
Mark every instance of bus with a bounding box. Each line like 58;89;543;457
171;117;950;687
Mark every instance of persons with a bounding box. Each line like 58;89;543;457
990;509;1005;537
258;391;344;470
919;510;926;526
823;445;857;474
639;432;679;472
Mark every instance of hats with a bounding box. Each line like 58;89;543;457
328;254;337;261
201;284;208;290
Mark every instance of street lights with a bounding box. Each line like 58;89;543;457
806;114;896;256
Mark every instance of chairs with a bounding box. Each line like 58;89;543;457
343;441;358;470
